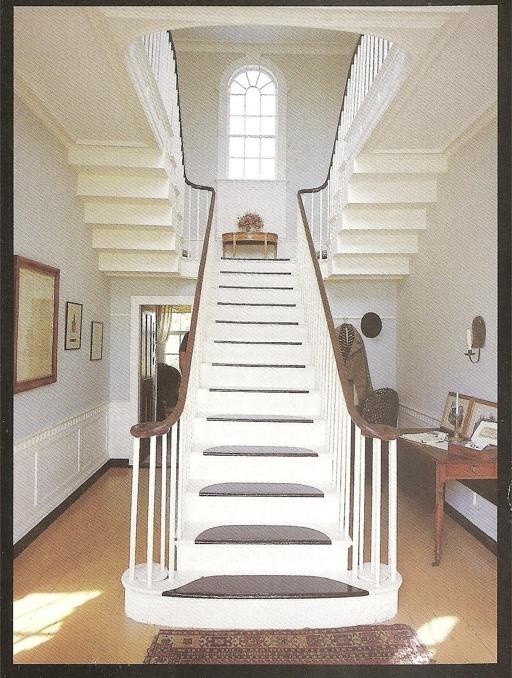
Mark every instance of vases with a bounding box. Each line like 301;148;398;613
247;225;257;232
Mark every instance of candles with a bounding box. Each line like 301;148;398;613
465;327;472;352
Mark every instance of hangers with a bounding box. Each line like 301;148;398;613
336;316;359;331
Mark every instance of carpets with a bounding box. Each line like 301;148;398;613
141;622;434;668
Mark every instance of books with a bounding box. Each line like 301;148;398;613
398;431;454;451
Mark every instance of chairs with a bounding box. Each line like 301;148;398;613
351;387;400;469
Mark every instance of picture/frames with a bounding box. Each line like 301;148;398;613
90;321;103;362
13;255;61;394
64;301;84;351
460;397;497;447
440;392;471;434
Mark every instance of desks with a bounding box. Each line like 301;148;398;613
222;233;278;260
390;426;496;568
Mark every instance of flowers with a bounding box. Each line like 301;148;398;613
237;212;264;228
